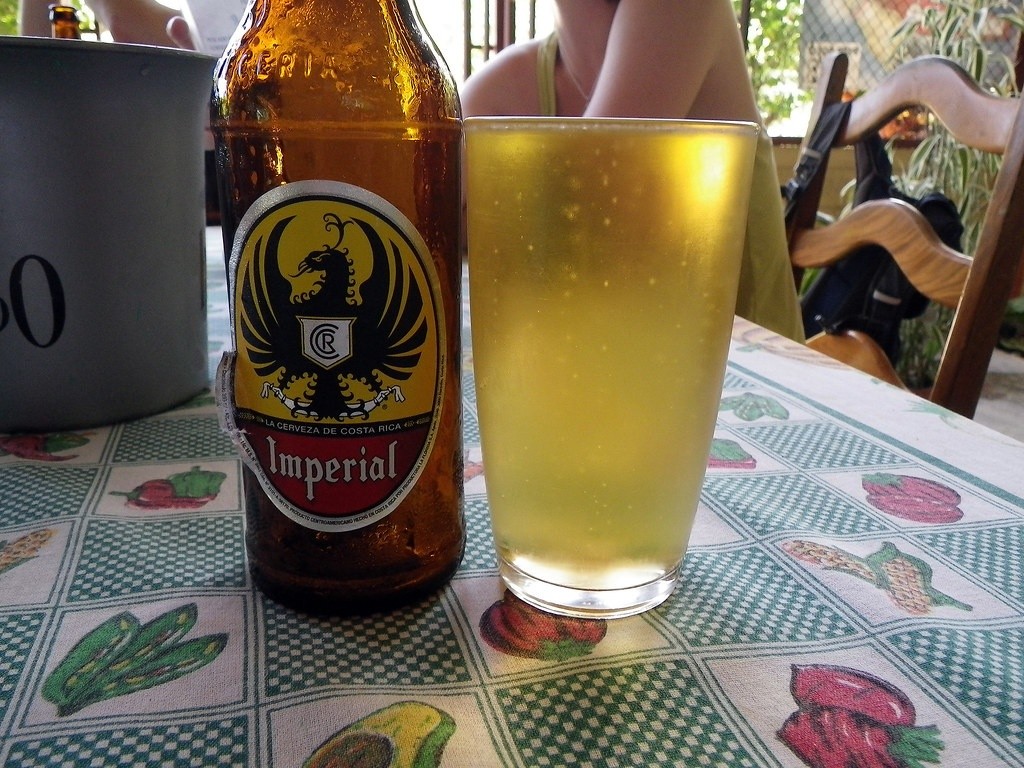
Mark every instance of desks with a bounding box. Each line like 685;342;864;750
1;222;1024;768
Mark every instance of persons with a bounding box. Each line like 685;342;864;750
166;0;806;344
18;0;204;52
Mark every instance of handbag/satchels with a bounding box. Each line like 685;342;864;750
777;99;965;369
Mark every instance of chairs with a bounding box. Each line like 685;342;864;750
776;50;1024;421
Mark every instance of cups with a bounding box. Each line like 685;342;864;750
461;114;761;619
0;33;213;436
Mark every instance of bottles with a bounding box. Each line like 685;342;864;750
49;4;81;39
210;0;467;620
